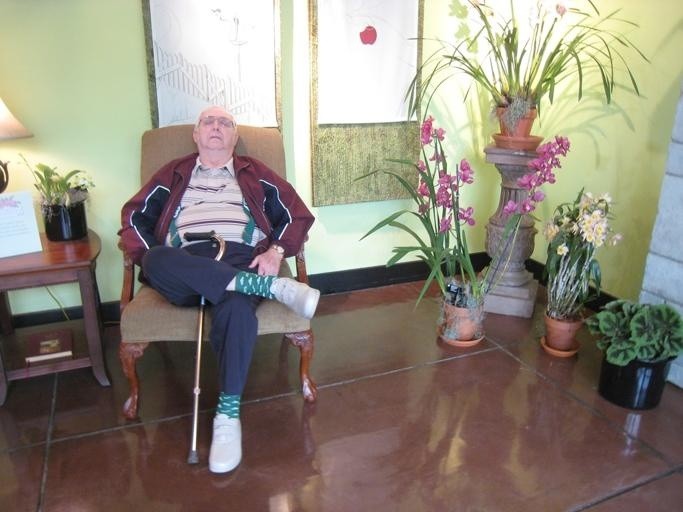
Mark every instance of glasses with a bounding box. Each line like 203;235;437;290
200;116;237;128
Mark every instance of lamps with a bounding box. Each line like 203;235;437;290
0;91;34;193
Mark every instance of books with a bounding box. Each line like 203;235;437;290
23;326;72;363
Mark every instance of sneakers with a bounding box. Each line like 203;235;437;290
208;413;242;474
269;277;321;320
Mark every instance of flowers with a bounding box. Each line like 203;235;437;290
352;115;572;317
403;0;656;126
542;185;626;319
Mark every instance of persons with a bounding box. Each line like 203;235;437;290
117;104;320;473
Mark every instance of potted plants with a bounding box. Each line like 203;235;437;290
583;296;681;411
26;159;95;241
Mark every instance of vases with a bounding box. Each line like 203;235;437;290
435;295;486;349
488;90;544;151
539;305;583;360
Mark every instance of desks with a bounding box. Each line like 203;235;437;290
0;225;112;408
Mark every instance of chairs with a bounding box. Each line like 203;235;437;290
117;124;319;421
